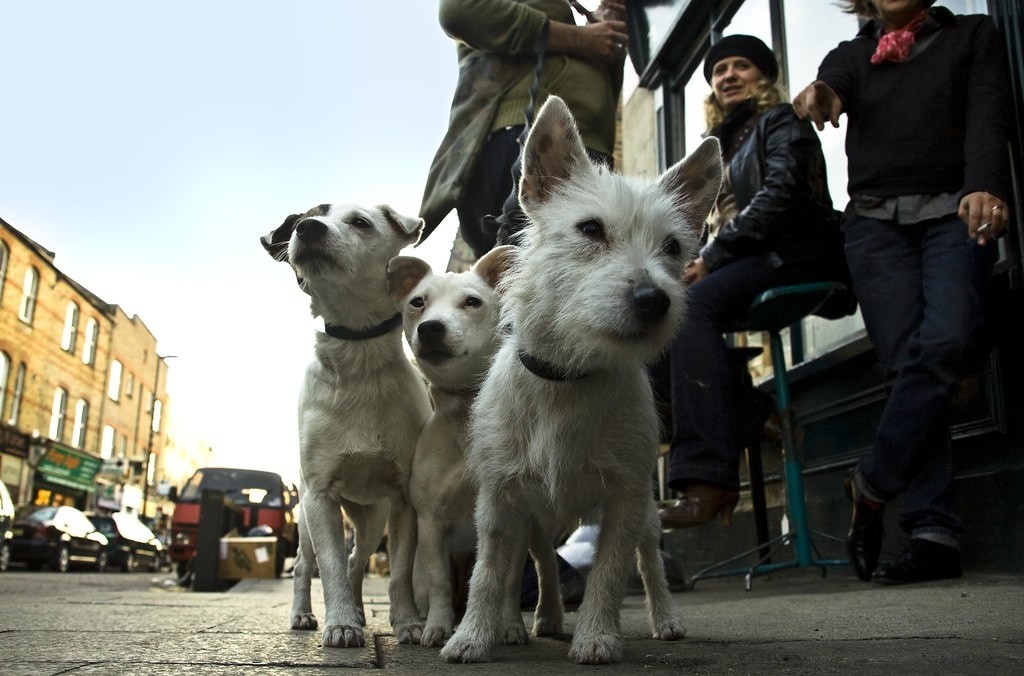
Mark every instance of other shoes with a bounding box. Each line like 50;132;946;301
517;550;587;614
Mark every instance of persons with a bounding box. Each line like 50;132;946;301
421;0;630;625
793;0;1013;584
632;36;834;529
150;507;172;572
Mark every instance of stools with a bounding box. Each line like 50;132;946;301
688;210;900;594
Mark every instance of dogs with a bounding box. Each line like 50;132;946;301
259;95;724;665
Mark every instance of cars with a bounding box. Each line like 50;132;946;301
0;478;17;573
9;503;111;574
84;511;163;572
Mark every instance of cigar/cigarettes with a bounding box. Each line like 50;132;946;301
976;221;993;234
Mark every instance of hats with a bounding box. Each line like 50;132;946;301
703;34;779;85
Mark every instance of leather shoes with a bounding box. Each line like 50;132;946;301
844;467;886;581
877;537;965;585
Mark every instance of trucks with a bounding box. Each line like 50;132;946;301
167;467;301;587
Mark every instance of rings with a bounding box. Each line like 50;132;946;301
991;204;1003;212
618;42;623;49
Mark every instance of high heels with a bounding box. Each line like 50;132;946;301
658;481;740;527
